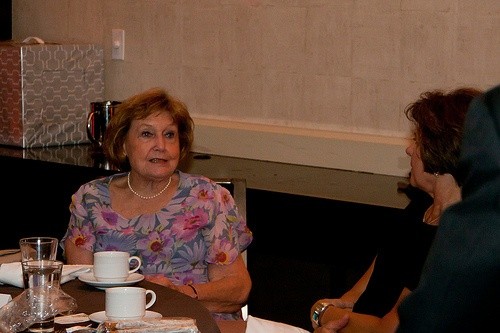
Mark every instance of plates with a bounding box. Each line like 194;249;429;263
79;273;144;290
89;310;162;324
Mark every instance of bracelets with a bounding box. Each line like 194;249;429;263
184;283;199;300
311;303;334;329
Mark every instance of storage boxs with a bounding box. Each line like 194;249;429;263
0;37;107;148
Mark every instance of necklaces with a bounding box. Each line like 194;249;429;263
128;171;171;200
424;205;440;224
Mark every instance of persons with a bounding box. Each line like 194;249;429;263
308;88;483;333
392;83;500;333
57;87;254;332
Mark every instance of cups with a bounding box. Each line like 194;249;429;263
94;251;141;281
105;287;156;320
19;237;58;308
21;259;63;333
86;100;123;146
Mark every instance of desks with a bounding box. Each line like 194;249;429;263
0;144;434;327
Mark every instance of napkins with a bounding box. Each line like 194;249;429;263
244;315;311;333
0;260;94;289
0;294;13;308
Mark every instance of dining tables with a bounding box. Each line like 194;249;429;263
0;264;247;333
